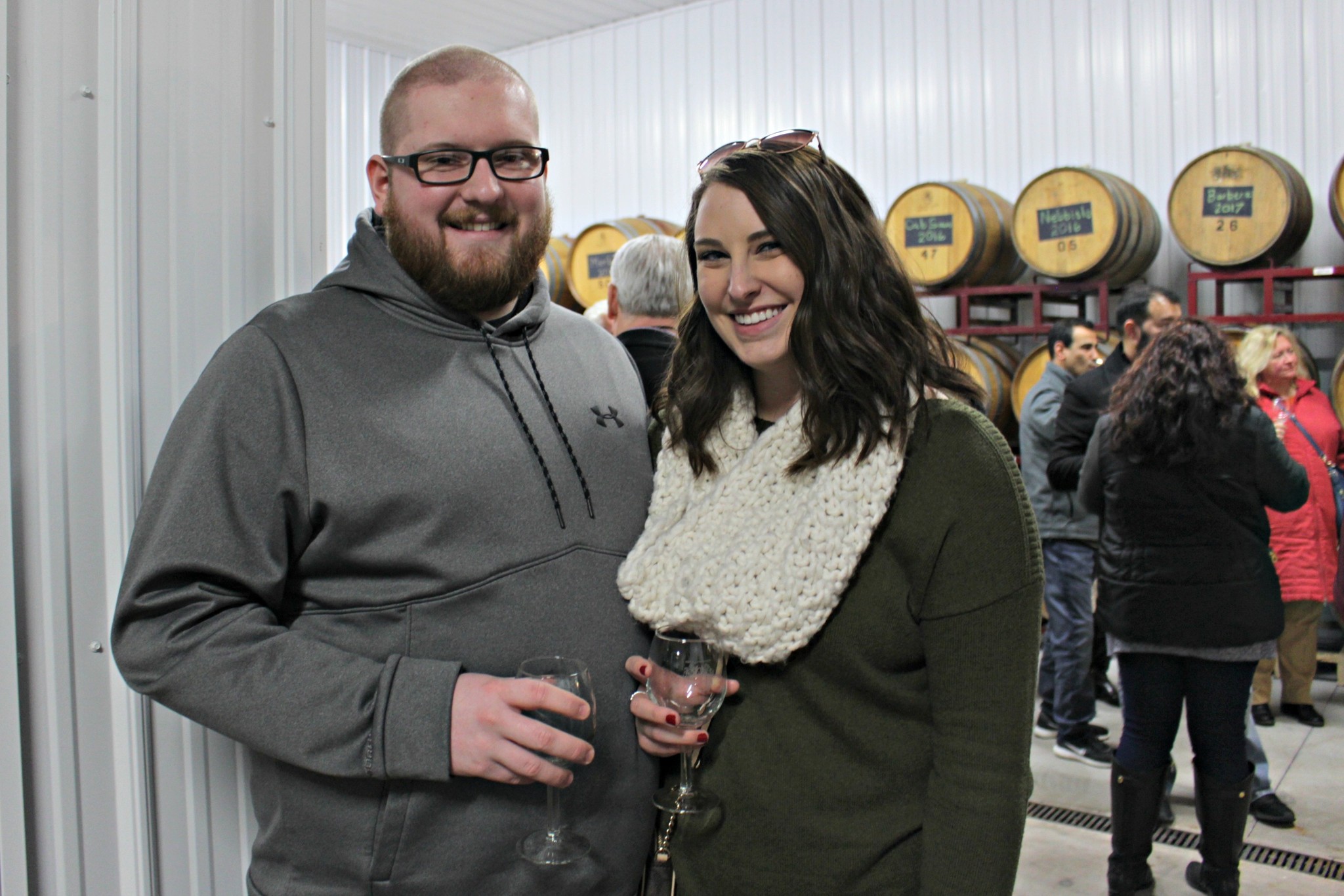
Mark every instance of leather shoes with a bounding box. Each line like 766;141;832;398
1280;698;1325;727
1250;702;1275;727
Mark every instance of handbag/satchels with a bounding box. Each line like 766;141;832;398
1328;467;1344;537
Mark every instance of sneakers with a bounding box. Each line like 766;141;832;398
1055;728;1117;768
1247;793;1294;826
1033;711;1059;740
1157;798;1176;823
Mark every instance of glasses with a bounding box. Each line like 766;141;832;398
698;129;827;182
383;144;552;185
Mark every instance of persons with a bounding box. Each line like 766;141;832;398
108;48;665;896
598;129;1343;896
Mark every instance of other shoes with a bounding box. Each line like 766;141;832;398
1109;866;1155;896
1095;679;1122;709
1185;861;1217;896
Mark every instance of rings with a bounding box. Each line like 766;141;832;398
630;691;648;704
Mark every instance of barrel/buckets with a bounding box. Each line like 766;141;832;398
1168;145;1313;271
927;334;1025;433
537;236;585;314
883;181;1029;292
1011;329;1123;424
1329;155;1344;241
674;227;687;240
566;218;684;309
1010;167;1161;284
1330;345;1344;430
1218;323;1319;391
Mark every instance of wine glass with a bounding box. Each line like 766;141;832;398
643;624;727;814
513;656;595;865
